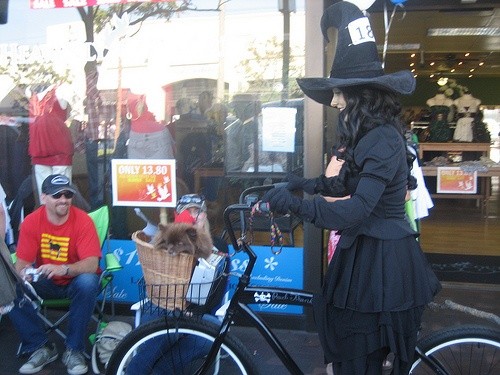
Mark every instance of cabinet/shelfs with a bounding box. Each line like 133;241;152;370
417;142;500;225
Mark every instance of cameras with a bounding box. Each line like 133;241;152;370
25;269;43;282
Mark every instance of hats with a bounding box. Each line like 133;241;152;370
176;194;207;214
295;2;416;107
42;174;76;196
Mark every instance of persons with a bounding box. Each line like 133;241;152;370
249;2;442;375
322;145;348;269
0;184;17;317
453;91;483;142
117;65;177;165
124;193;230;375
426;95;453;142
74;63;126;240
7;173;103;375
24;74;74;204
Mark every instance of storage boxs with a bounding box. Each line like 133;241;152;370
184;248;226;306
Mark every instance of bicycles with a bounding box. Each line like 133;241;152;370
103;183;500;374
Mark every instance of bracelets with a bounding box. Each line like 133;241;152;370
62;265;69;275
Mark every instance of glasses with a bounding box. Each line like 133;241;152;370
53;191;74;199
176;195;203;204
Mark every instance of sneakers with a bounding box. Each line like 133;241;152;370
18;342;59;374
62;349;88;375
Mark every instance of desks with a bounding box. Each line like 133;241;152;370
192;167;224;195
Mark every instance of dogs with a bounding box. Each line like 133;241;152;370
155;223;212;258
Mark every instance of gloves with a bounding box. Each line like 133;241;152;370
279;171;317;195
261;186;302;215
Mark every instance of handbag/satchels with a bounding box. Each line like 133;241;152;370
96;320;134;369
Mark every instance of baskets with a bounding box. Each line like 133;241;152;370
135;261;230;317
132;230;197;311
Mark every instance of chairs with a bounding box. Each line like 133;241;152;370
10;205;124;362
